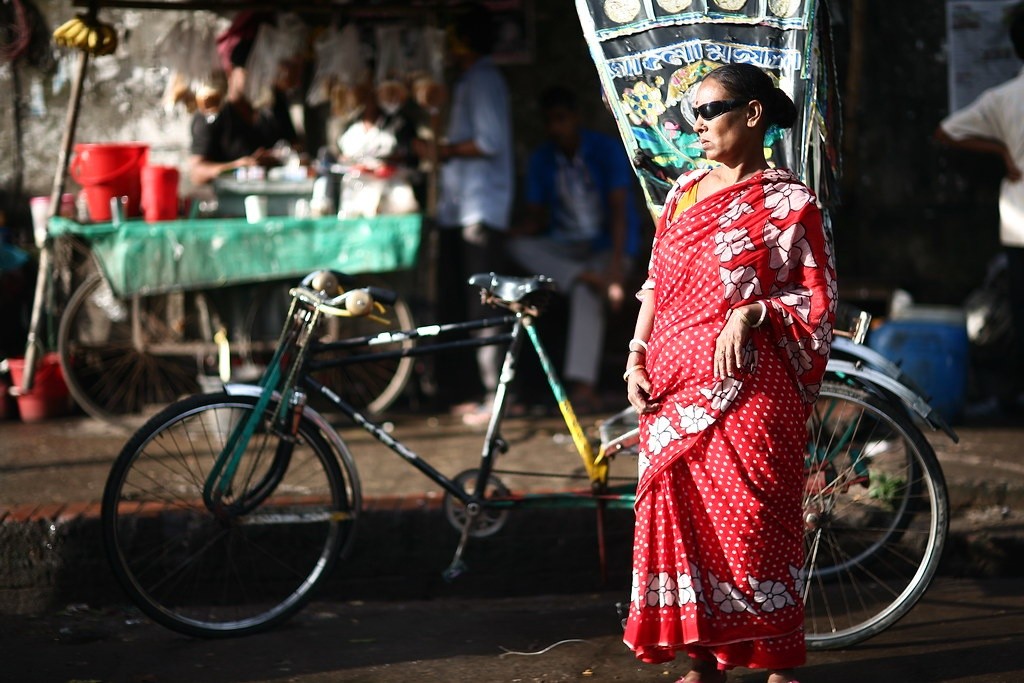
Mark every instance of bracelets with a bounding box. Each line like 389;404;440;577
733;309;752;329
753;301;767;328
627;350;645;357
628;337;648;352
623;364;646;382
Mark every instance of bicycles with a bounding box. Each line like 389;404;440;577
59;237;417;434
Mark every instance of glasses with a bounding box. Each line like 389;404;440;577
692;99;748;122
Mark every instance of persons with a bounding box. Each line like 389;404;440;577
621;61;838;683
504;75;643;420
173;0;515;431
933;2;1022;427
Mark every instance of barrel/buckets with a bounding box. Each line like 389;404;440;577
872;324;983;416
136;165;180;222
0;353;76;424
68;142;151;223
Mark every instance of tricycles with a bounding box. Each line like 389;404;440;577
99;258;955;641
